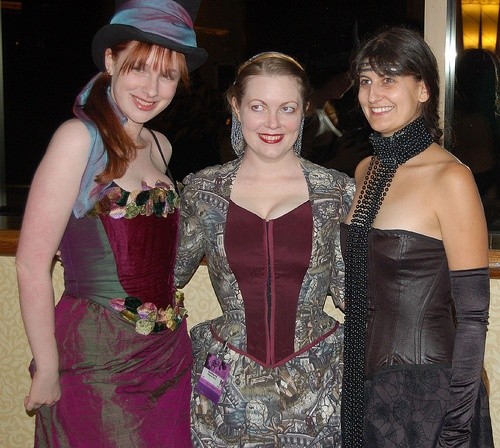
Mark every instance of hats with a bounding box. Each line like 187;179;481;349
92;0;208;75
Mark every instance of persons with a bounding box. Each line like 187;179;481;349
17;2;209;447
341;28;495;448
172;53;356;447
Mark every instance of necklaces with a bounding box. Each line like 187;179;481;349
341;117;436;447
